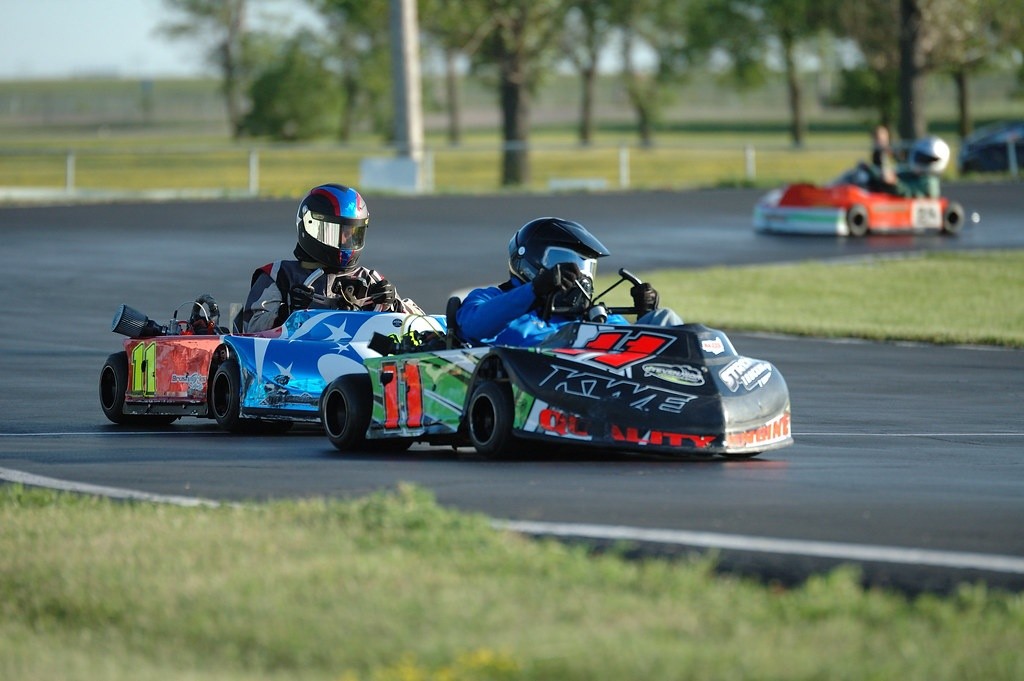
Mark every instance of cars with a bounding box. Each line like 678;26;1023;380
954;118;1024;176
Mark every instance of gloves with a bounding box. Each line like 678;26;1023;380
279;283;315;310
369;279;397;305
534;262;582;297
630;282;660;315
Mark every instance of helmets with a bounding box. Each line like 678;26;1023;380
909;134;951;174
295;183;369;269
508;216;599;287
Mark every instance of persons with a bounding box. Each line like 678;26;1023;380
243;183;401;333
456;217;659;348
872;124;950;198
